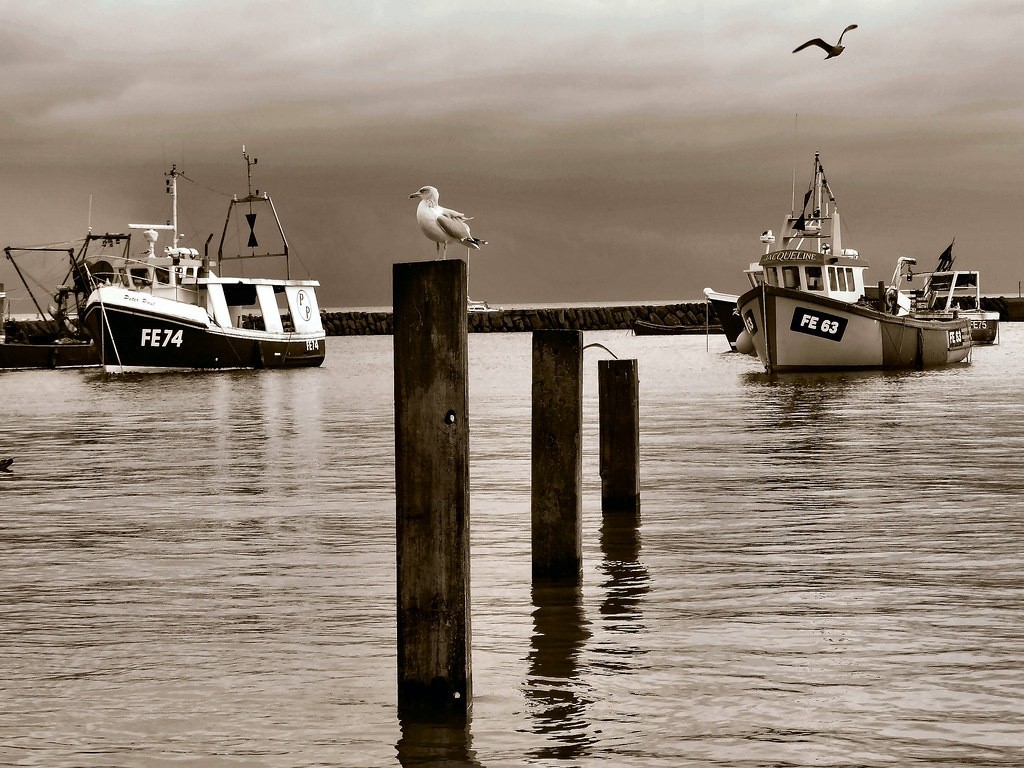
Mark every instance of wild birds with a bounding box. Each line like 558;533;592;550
793;24;859;60
408;185;489;260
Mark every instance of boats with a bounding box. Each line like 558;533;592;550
82;144;327;381
467;296;500;312
701;235;1001;354
633;319;726;337
736;113;975;376
0;230;132;371
